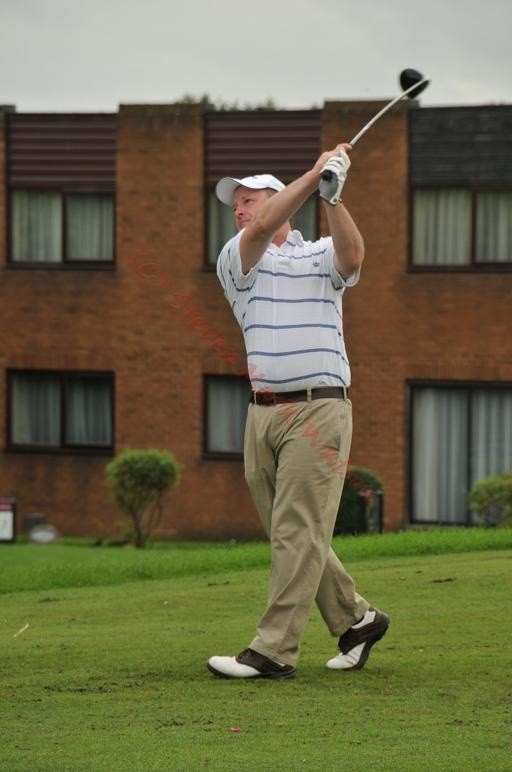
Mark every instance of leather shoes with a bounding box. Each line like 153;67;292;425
206;647;296;682
326;604;389;671
248;387;344;405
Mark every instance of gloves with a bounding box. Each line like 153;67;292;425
319;150;351;205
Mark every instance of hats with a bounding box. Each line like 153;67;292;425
215;174;286;207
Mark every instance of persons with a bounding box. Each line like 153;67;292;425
205;142;392;679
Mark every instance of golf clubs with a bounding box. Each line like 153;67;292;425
320;68;430;180
